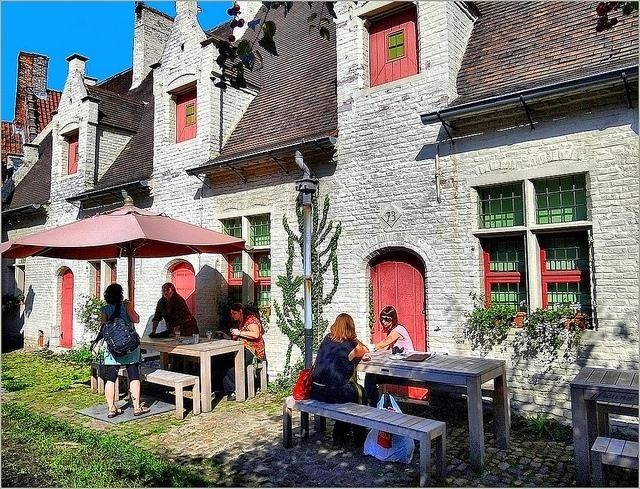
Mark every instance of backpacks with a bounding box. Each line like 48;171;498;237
88;303;141;358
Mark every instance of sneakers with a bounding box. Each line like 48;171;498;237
332;432;350;444
354;432;368;448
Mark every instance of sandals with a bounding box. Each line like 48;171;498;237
133;406;150;416
107;408;122;418
226;390;236;401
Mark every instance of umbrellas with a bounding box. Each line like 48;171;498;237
2;196;245;330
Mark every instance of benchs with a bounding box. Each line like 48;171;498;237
596;400;640;437
91;362;201;420
141;352;268;397
282;395;446;487
362;376;495;405
591;436;640;481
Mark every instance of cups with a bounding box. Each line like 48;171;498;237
174;330;212;344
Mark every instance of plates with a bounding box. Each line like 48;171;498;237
388;352;409;361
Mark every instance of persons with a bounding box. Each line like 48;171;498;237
151;282;199;373
100;284;150;417
310;313;371;441
365;306;415;406
217;302;265;401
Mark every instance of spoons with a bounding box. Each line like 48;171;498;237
425;351;437;361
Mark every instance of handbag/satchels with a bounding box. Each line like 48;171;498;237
292;368;313;400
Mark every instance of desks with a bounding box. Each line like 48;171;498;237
355;345;510;474
569;367;640;488
98;327;246;412
